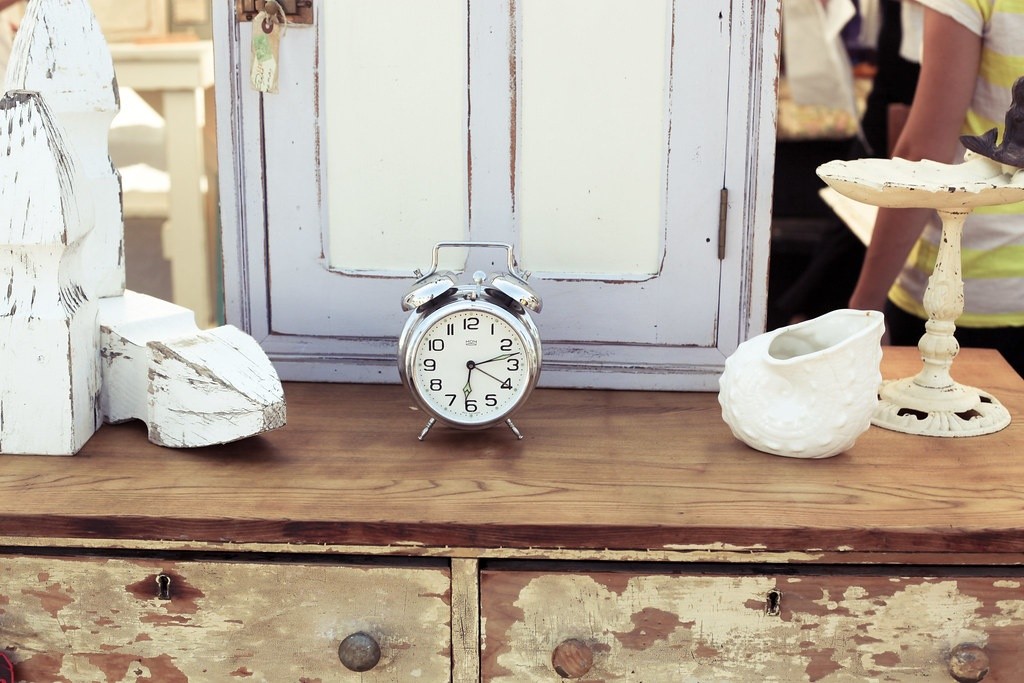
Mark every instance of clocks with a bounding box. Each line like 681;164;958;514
395;243;546;444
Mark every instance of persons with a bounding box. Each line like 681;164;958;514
851;0;923;161
846;0;1024;382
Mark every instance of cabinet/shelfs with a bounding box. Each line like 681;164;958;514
1;349;1021;683
211;0;775;398
